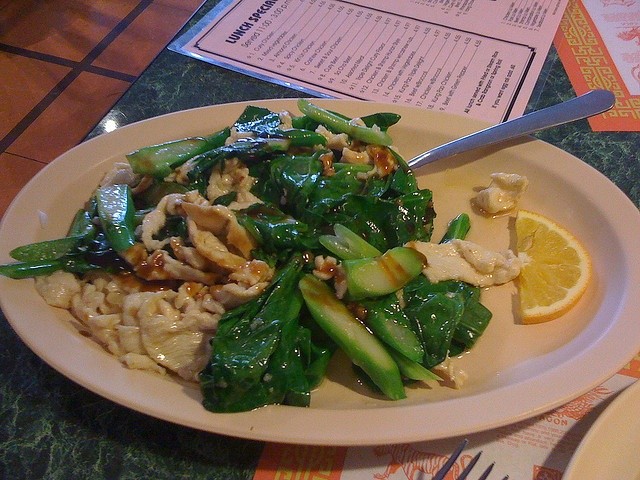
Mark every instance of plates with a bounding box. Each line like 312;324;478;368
0;97;640;447
561;381;640;480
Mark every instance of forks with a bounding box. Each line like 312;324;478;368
430;439;512;480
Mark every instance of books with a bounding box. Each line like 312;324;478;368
167;0;571;137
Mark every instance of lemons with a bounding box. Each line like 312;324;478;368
515;209;594;324
